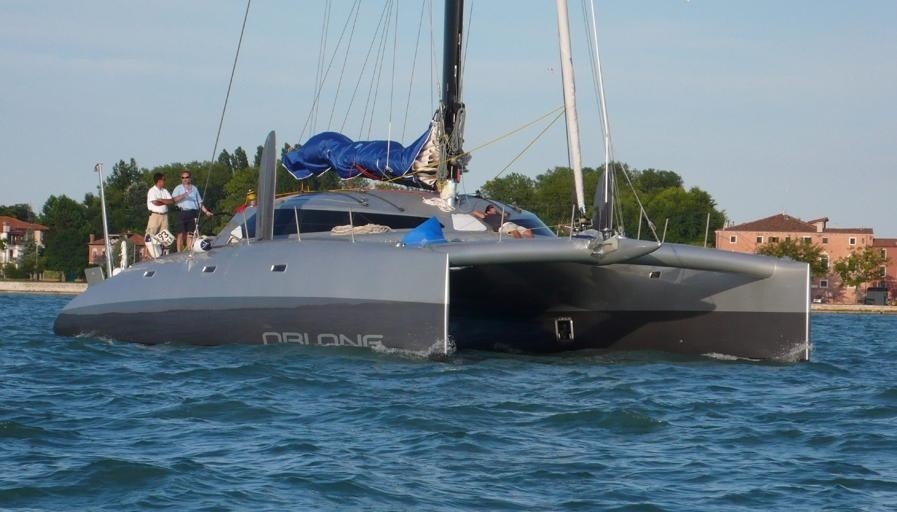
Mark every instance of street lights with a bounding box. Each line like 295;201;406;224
92;162;114;279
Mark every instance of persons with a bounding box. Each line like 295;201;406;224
142;173;176;259
471;205;534;239
172;170;213;254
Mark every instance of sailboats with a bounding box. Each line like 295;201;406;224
51;1;810;365
